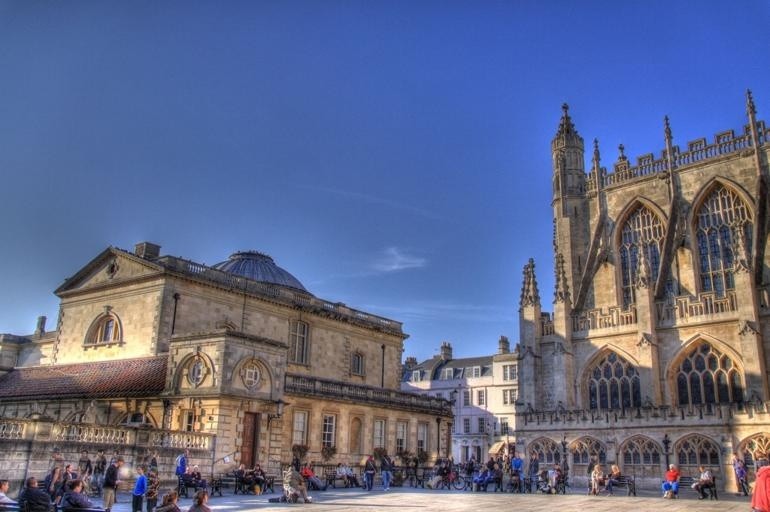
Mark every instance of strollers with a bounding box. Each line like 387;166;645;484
503;470;521;494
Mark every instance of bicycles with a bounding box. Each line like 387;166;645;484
441;471;466;490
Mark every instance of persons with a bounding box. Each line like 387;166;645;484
391;450;571;494
600;464;621;496
380;454;392;491
753;450;769;474
690;465;713;499
591;465;604;496
363;455;378;491
291;454;300;471
751;449;770;512
732;452;753;495
0;446;212;512
662;464;680;498
235;463;362;503
